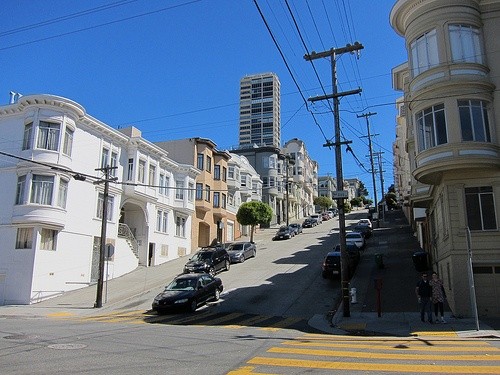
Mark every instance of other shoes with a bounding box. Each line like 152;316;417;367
435;316;438;321
441;316;444;322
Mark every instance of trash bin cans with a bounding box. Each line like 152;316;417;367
413;252;427;273
375;253;385;270
371;220;378;228
379;219;384;227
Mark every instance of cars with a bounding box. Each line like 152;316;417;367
302;208;339;228
183;244;231;277
353;225;372;239
333;242;361;265
152;273;223;316
288;223;303;235
224;241;256;264
357;218;372;230
276;226;295;240
322;251;354;278
345;232;365;250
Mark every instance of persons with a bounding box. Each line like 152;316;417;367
429;272;448;323
415;272;434;324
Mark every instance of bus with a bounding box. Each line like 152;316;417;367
369;205;380;220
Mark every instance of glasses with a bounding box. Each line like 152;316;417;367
423;276;427;277
